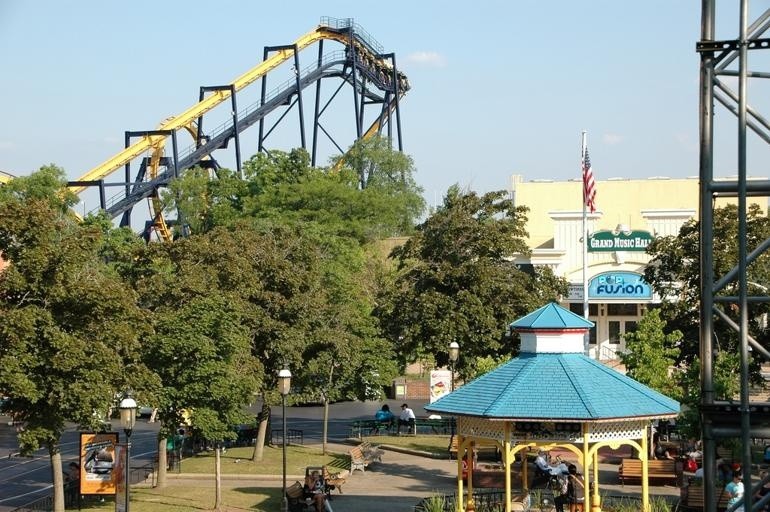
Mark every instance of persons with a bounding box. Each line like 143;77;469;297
368;404;394;436
394;403;416;436
756;469;770;511
723;471;745;511
652;439;703;460
694;462;744;484
535;448;569;476
554;463;586;511
302;471;335;512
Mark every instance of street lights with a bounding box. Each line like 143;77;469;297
115;390;141;512
446;335;460;391
276;360;293;512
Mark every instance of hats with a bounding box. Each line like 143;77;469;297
400;404;408;408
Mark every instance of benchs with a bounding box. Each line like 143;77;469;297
447;434;503;462
321;465;345;495
282;480;317;512
686;486;731;512
63;478;80;502
618;458;678;487
404;416;457;435
348;418;394;438
695;449;735;469
348;440;384;476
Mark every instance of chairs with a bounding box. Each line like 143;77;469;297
511;492;531;512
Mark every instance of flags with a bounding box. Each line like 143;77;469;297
581;129;597;215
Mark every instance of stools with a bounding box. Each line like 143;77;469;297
570;503;583;512
